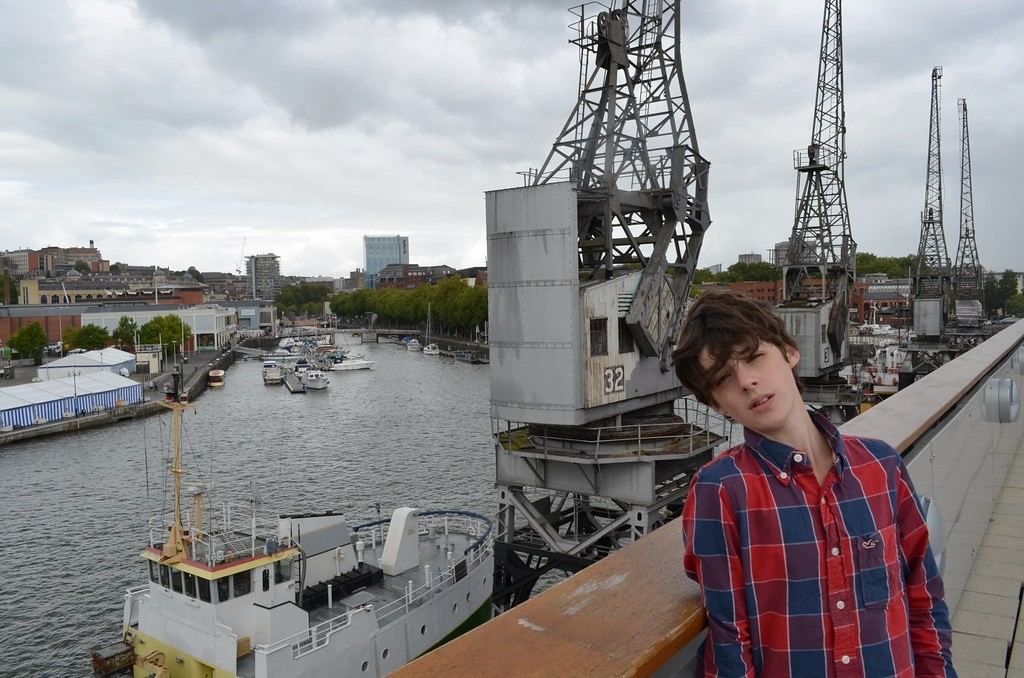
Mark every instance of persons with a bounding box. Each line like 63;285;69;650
674;292;956;678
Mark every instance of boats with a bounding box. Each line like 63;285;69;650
423;343;439;355
262;310;377;394
455;353;479;364
208;369;225;387
406;339;421;350
78;356;496;678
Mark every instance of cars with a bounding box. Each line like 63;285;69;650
858;315;1022;338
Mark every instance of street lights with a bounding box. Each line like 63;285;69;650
164;343;168;370
171;340;176;365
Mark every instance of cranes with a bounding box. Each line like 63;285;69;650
910;65;952;342
770;0;857;381
947;98;989;327
481;0;715;432
